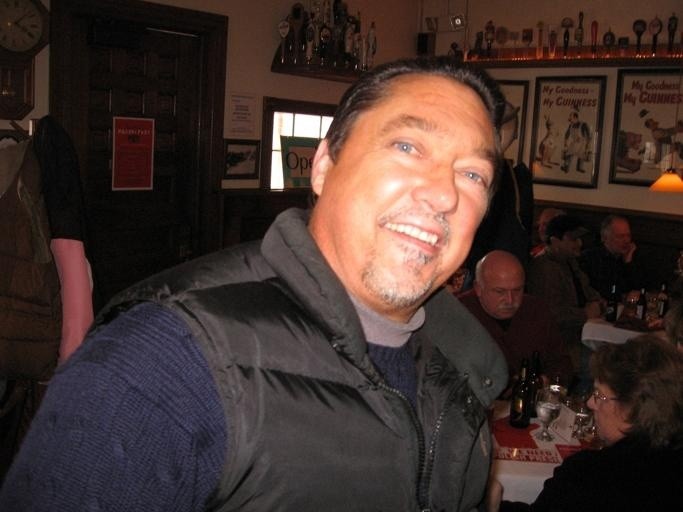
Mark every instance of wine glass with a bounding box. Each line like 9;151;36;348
536;403;560;442
575;413;591;440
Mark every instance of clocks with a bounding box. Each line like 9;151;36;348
0;0;51;120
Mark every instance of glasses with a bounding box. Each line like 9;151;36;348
593;386;620;404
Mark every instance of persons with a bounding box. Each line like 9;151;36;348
530;334;683;512
0;57;512;510
540;104;683;172
457;207;657;391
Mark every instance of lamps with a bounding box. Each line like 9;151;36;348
647;73;682;192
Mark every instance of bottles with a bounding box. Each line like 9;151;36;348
635;289;647;323
511;351;538;428
658;284;668;318
604;285;618;323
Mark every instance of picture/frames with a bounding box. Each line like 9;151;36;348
222;138;260;180
483;79;529;169
608;69;683;186
526;76;606;189
